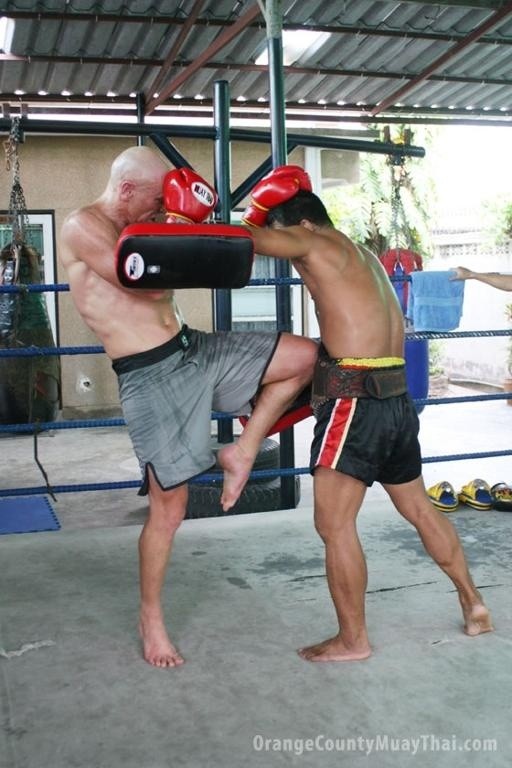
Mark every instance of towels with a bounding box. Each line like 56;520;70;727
404;270;465;332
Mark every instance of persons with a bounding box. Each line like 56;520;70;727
58;146;319;668
243;188;494;661
449;266;512;291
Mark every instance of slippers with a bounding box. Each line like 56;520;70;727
427;479;511;512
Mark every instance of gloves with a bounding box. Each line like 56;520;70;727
162;166;220;224
242;165;312;229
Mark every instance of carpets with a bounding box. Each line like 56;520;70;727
1;495;63;535
